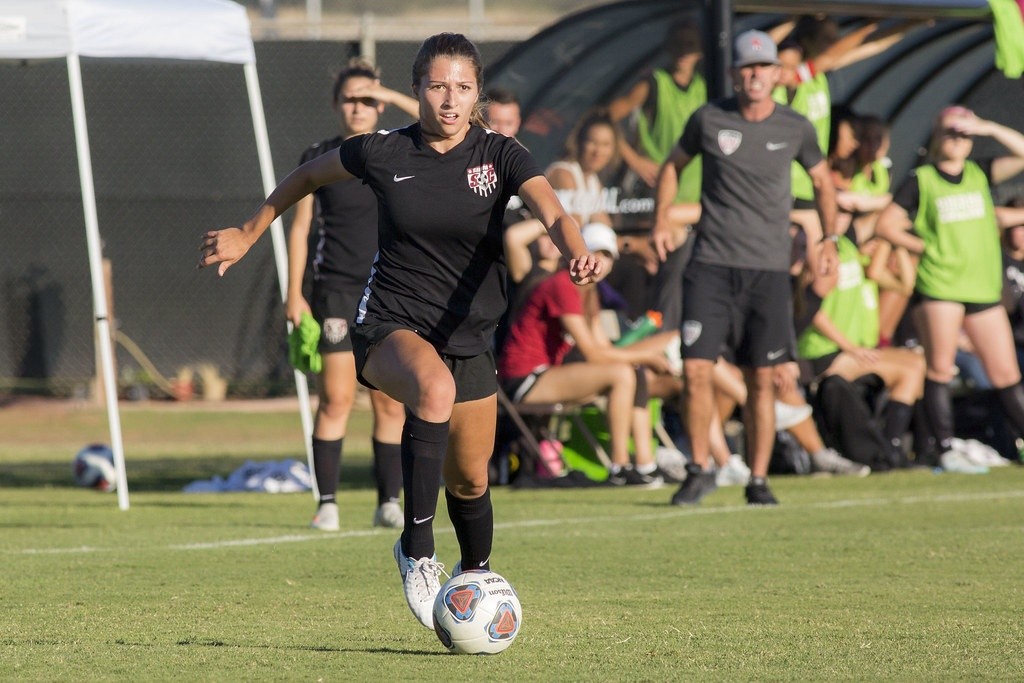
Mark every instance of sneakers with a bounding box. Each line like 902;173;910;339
452;561;462;577
393;539;451;631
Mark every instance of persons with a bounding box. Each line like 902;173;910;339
284;60;420;533
194;33;603;630
484;9;752;489
651;29;840;507
765;14;1024;477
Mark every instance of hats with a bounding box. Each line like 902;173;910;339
734;29;783;68
583;222;620;259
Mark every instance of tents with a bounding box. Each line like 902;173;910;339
0;0;316;509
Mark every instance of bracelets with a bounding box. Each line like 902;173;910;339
819;235;838;249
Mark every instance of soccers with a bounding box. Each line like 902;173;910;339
73;443;117;493
432;568;523;657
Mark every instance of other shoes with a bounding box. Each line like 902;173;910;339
745;483;778;505
717;455;750;486
939;450;988;474
808;448;870;478
775;403;812;430
672;463;717;506
373;503;404;529
314;503;340;532
612;466;677;487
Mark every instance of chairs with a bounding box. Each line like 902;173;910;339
491;378;627;492
